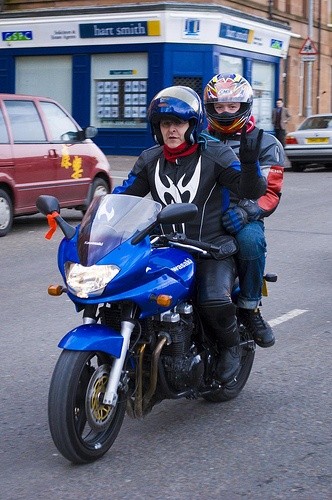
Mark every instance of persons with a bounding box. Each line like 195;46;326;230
271;98;291;147
97;73;285;383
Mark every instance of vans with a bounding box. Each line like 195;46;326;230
0;95;114;239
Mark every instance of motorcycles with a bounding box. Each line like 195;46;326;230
34;193;279;464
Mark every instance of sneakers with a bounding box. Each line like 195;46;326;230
240;309;275;347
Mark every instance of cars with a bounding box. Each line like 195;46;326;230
284;113;332;169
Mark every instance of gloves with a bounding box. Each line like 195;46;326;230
238;124;264;163
222;205;248;233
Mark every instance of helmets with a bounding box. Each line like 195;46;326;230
203;73;254;134
146;85;204;144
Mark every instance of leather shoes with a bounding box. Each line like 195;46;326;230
215;340;241;383
